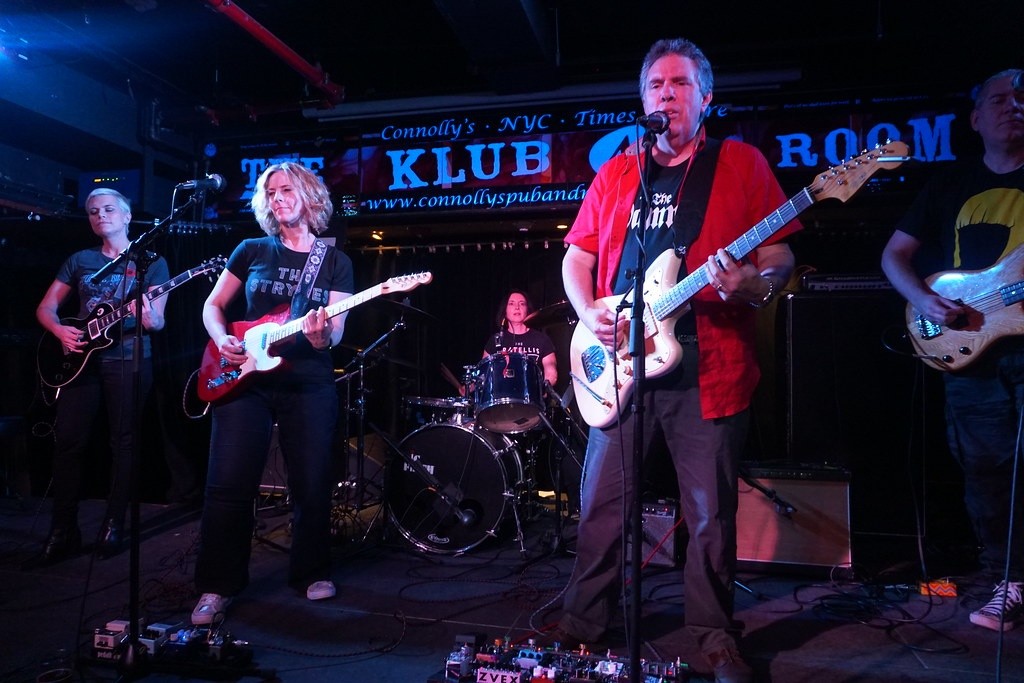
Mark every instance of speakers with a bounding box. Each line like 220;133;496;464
258;423;288;495
733;464;854;581
788;290;925;538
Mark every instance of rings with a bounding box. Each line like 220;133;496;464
935;320;940;322
716;284;723;291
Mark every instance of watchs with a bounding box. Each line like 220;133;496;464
750;275;774;309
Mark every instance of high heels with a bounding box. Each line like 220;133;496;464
18;515;82;572
94;509;124;558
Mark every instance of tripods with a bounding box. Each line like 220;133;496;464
331;319;464;564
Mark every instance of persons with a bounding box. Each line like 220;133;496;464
542;41;801;683
462;291;560;400
191;161;356;623
17;188;169;573
880;65;1023;633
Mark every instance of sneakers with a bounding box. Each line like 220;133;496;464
968;580;1024;631
190;590;234;625
306;580;336;600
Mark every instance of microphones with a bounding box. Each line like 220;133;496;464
637;111;670;134
458;510;476;529
501;319;506;338
775;503;797;512
175;174;222;190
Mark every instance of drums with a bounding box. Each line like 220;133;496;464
384;353;546;555
197;270;433;405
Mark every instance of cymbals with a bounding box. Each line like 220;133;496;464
522;300;571;324
373;296;437;330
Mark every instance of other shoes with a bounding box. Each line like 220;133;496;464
537;628;578;653
701;647;754;683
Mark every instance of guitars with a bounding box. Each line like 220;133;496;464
36;256;230;387
904;243;1024;374
568;138;913;429
